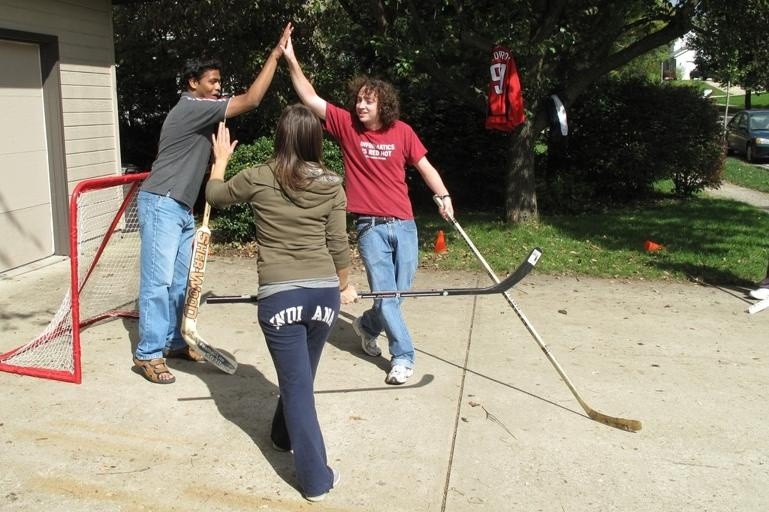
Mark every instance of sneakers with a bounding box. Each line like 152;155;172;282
352;317;382;357
386;364;414;384
306;469;339;501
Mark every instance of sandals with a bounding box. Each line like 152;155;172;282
133;356;176;383
165;344;206;362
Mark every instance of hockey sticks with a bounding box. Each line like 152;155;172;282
207;246;543;303
180;145;238;374
433;194;643;432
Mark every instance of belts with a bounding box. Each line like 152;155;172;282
355;215;396;244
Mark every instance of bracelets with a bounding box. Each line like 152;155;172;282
340;284;350;293
436;194;450;200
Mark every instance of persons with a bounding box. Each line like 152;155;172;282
133;22;294;383
205;103;360;503
280;37;455;385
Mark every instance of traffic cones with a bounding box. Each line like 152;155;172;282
434;231;448;252
645;240;663;253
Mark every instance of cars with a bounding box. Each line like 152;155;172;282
725;108;769;163
